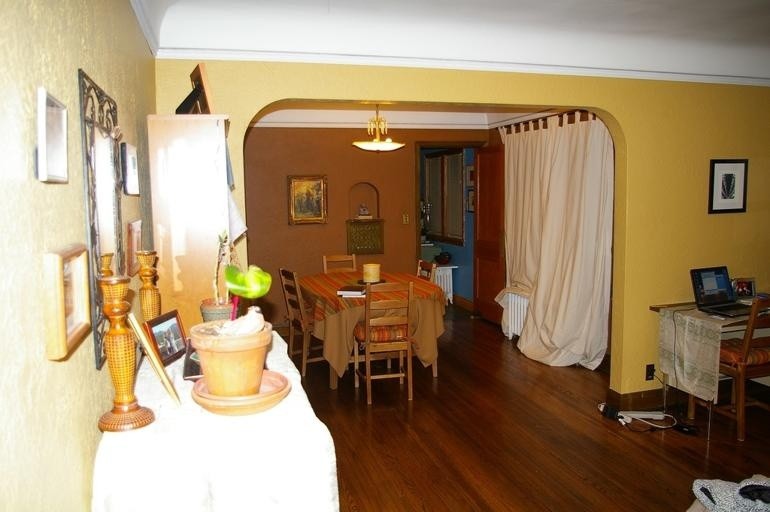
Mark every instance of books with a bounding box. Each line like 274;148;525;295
337;286;366;299
184;339;267;382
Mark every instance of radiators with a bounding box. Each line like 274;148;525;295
506;291;534;341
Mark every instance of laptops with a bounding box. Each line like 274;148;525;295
688;267;752;318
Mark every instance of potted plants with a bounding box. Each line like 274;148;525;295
188;257;292;417
199;225;240;323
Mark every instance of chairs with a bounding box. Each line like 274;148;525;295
352;279;415;405
687;296;770;442
321;252;357;274
391;259;436;387
277;267;349;378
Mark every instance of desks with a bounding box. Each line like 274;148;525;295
295;268;447;391
435;265;459;304
90;330;342;511
649;293;769;421
420;246;443;263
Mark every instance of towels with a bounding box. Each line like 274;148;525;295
228;183;249;245
226;136;238;192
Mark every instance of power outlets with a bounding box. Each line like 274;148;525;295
645;362;655;380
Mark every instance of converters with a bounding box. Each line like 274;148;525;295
673;423;691;434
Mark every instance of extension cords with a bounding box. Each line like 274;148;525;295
618;409;666;422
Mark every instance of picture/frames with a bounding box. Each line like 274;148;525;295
174;62;217;115
120;142;142;197
468;190;475;214
128;218;144;280
287;173;330;225
43;245;92;363
33;85;70;185
465;165;475;188
708;157;748;215
732;276;756;297
142;306;189;367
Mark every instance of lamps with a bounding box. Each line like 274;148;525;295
351;102;407;155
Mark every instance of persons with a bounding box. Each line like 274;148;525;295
163;327;179;357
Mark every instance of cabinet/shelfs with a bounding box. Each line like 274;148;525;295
145;113;232;336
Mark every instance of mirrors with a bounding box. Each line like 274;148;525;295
76;67;127;372
